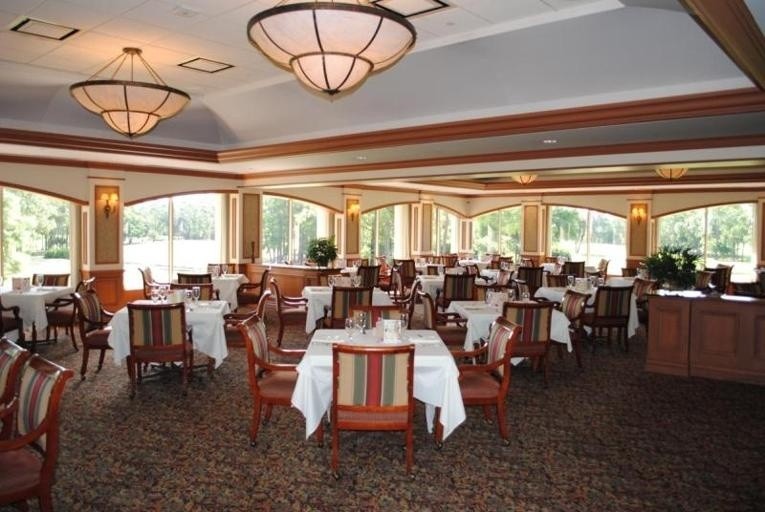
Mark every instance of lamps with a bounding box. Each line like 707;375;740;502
512;174;538;186
70;47;190;139
631;208;644;225
100;192;118;218
246;0;416;96
655;168;689;180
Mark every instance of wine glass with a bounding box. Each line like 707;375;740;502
485;286;530;311
327;260;362;292
35;273;45;292
211;264;228;277
567;273;607;293
344;312;409;345
150;285;201;311
